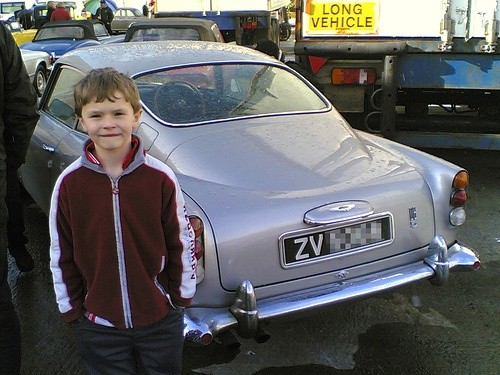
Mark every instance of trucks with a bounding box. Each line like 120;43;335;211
292;0;500;154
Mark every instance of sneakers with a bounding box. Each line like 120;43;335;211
16;268;42;293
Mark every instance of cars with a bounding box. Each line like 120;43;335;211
14;39;482;345
0;2;280;99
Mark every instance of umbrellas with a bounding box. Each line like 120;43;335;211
83;0;119;20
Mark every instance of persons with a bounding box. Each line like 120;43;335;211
0;22;40;271
94;0;113;35
143;1;150;16
49;68;197;375
46;1;71;21
18;5;33;29
251;39;324;98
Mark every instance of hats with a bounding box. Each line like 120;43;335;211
101;0;106;3
57;2;65;8
